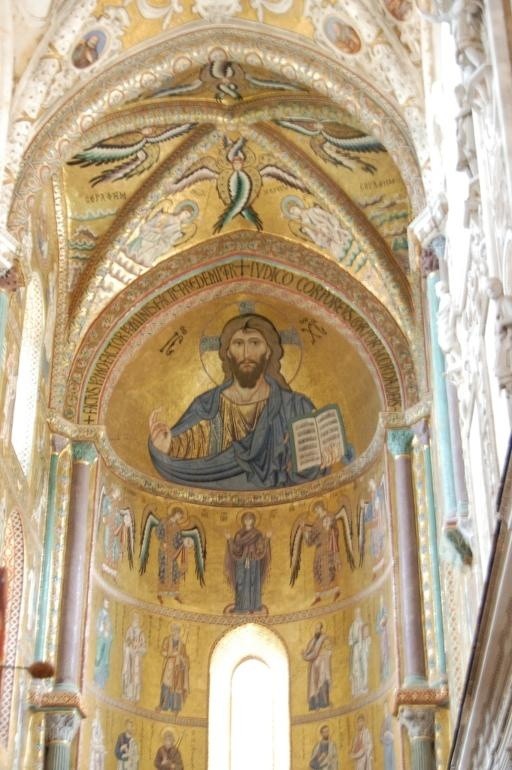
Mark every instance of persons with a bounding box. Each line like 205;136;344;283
288;201;351;260
454;84;477;171
149;314;343;491
486;276;512;393
72;35;99;69
126;206;195;267
331;22;361;55
434;281;451;354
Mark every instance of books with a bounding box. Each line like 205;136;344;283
287;402;349;474
